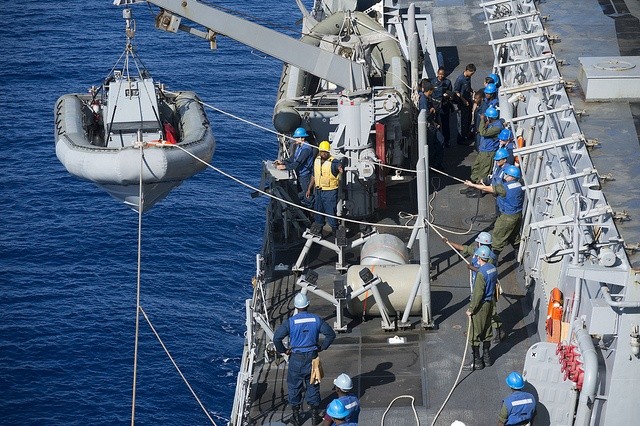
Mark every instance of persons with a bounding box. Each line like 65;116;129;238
274;127;316;218
442;232;498;338
273;293;336;426
305;140;345;235
326;398;358;426
318;373;360;426
475;73;522;259
462;246;498;370
496;372;537;426
417;60;473;170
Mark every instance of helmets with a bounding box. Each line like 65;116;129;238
498;128;513;141
494;148;510;160
333;372;353;393
489;74;501;85
293;127;309;137
319;140;331;151
326;399;350;418
506;372;525;389
476;245;492;260
504;165;521;178
484;82;498;94
485;106;499;120
294;293;310;309
475;232;493;244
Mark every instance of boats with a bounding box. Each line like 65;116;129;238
53;91;215;213
272;10;410;133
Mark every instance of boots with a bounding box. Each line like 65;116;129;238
288;407;301;425
311;407;323;425
462;345;484;369
480;341;492;366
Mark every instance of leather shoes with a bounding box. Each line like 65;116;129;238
467;189;483;198
460;186;475;193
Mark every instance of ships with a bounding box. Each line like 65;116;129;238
232;0;640;426
